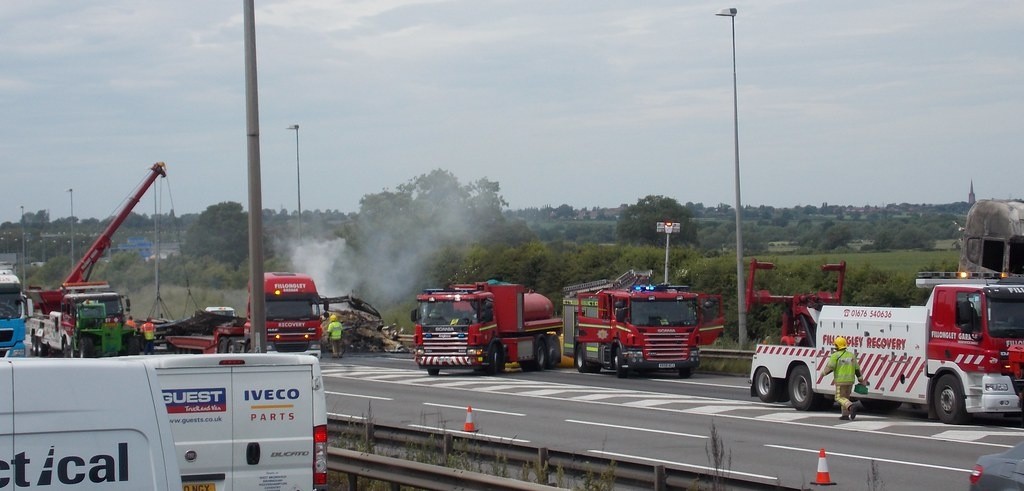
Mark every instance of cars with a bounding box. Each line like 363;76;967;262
966;438;1024;491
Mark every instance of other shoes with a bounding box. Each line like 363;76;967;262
840;415;850;420
851;404;858;419
333;352;342;358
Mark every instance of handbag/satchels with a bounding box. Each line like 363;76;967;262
854;380;869;395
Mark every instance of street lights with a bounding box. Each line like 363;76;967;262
67;188;74;272
286;125;306;275
714;7;748;352
20;204;26;292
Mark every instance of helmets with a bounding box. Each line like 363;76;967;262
330;315;336;321
833;337;848;350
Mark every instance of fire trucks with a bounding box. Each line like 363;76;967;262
409;280;565;377
559;266;726;379
746;255;1024;424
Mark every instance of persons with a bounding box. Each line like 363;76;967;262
139;317;157;355
326;314;345;358
124;315;138;336
820;337;868;421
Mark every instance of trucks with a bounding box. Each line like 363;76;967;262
0;268;33;359
167;270;331;360
27;282;145;357
0;353;331;491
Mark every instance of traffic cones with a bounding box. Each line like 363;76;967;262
808;448;837;486
460;406;480;433
